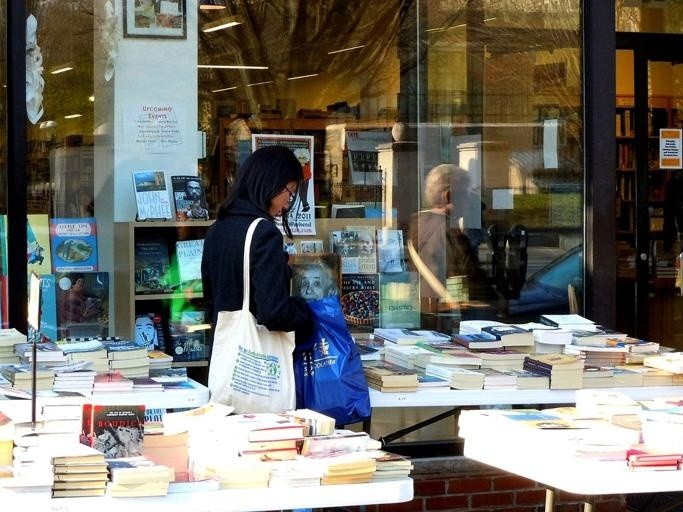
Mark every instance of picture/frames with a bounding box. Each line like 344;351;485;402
122;0;187;41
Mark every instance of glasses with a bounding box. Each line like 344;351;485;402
286;186;295;203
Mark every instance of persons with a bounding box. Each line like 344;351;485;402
200;144;314;390
409;163;500;321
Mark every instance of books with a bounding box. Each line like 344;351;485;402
353;314;682;392
285;227;420;330
132;171;207;221
0;214;413;501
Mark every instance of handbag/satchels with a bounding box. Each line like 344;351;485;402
208;310;296;413
296;296;370;425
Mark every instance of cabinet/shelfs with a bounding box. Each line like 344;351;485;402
110;216;217;368
529;92;682;352
217;117;383;207
